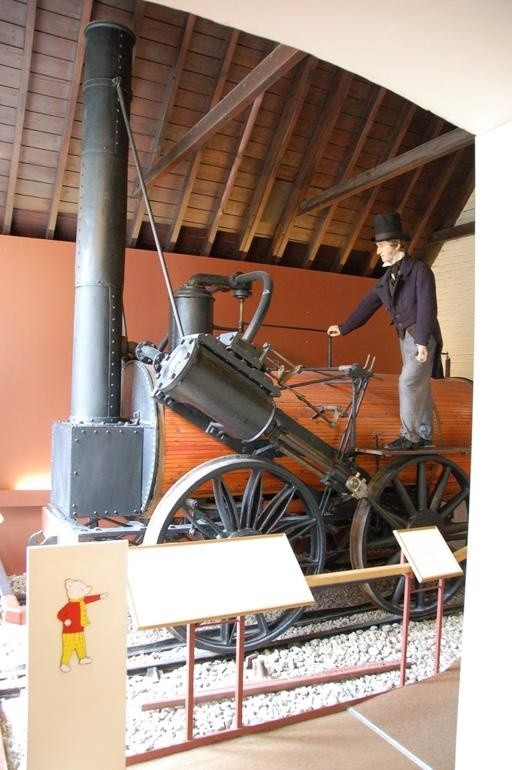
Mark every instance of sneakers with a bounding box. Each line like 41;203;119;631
384;436;435;451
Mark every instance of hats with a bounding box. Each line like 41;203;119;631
369;213;413;244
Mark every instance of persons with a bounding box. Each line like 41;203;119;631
326;211;448;450
